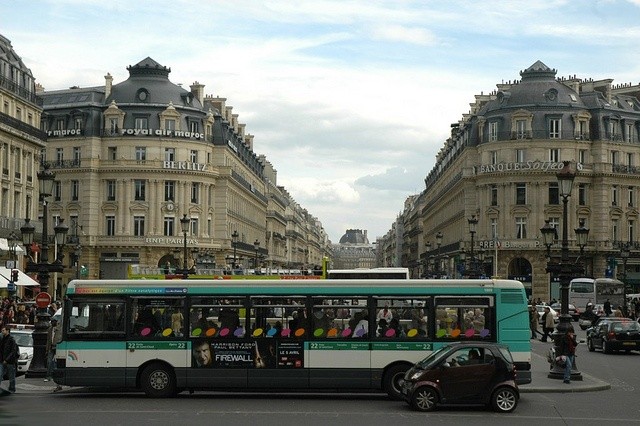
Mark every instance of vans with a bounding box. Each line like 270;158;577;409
6;323;34;329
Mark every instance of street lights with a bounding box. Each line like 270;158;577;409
618;241;630;317
180;213;190;277
7;231;18;269
254;239;260;258
468;214;478;278
436;231;443;263
539;159;590;379
232;230;239;263
425;240;431;257
21;161;69;379
74;222;86;279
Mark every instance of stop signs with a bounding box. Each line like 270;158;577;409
35;292;52;308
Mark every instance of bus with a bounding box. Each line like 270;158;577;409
49;277;534;398
569;278;596;307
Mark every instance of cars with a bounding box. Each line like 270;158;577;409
529;304;559;324
552;302;580;322
588;320;640;353
2;330;32;373
401;340;521;413
593;302;617;317
587;316;633;335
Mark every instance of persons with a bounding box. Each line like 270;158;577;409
312;302;330;321
348;318;356;331
472;309;485;333
537;298;542;304
164;261;171;275
0;294;63;328
539;308;555;342
560;326;577;384
297;309;305;318
467;350;480;364
622;297;640;322
292;311;298;320
550;299;557;306
43;316;59;383
170;308;184;335
530;306;538;339
0;327;20;392
377;319;388;333
376;305;392;322
586;300;595;312
604;299;612;316
388;318;401;336
192;339;211;368
414;303;423;322
218;304;240;331
